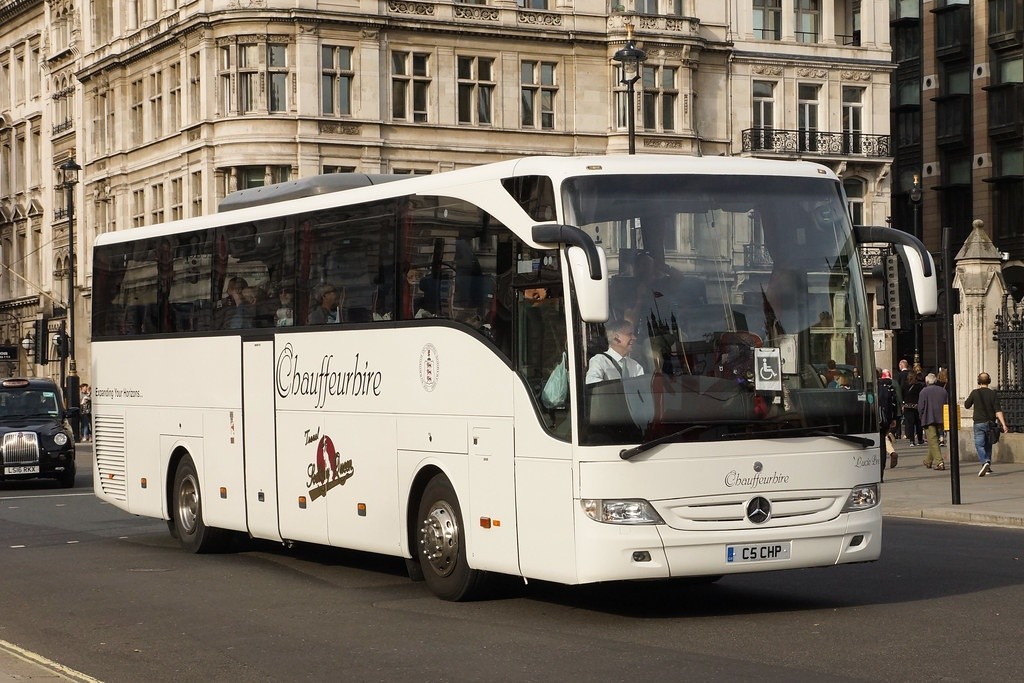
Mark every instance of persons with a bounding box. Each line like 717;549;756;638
934;368;948;447
80;384;92;442
918;374;948;471
876;360;927;468
585;319;655;421
964;373;1008;478
123;250;670;385
826;362;861;389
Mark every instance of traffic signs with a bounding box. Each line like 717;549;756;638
853;330;886;354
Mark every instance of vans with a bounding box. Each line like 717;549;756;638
813;363;862;390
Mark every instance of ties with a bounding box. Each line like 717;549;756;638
620;358;628;379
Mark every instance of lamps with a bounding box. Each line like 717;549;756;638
52;334;72;346
22;330;35;356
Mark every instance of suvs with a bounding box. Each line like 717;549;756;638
0;377;80;489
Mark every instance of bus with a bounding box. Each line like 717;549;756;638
88;148;940;603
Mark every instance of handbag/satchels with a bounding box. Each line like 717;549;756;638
541;351;567;408
989;421;1000;444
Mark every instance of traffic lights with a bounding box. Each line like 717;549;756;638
32;319;48;366
56;328;69;359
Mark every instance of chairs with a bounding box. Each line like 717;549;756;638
611;274;708;308
114;243;482;336
579;317;610;372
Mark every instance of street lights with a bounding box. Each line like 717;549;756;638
59;147;83;443
611;23;650;248
907;174;926;373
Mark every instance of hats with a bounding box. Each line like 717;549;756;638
882;369;891;379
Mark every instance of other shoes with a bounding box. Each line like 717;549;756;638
979;463;989;477
889;452;897;469
933;462;945;471
939;441;945;447
924;459;931;468
